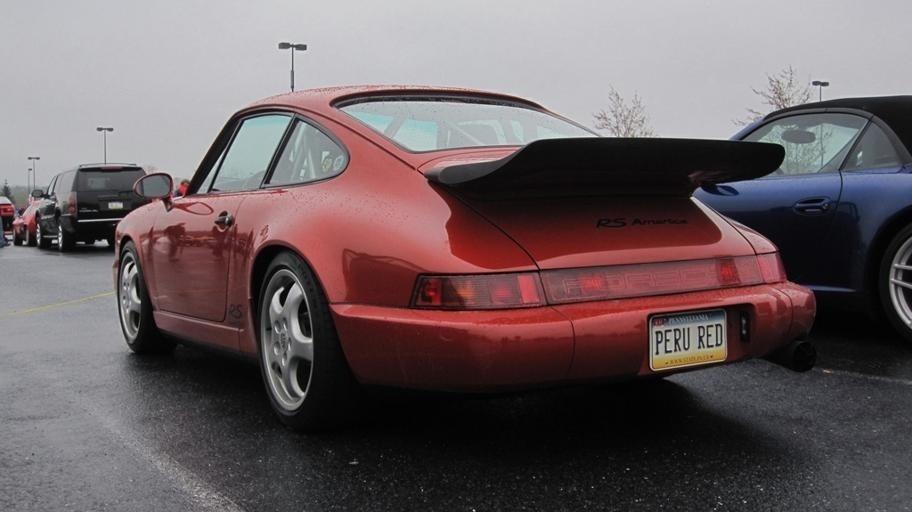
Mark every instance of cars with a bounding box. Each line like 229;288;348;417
111;82;820;429
11;163;152;251
692;94;912;334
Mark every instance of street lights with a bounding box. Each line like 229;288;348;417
23;153;42;191
806;74;834;100
276;37;308;94
94;123;116;164
